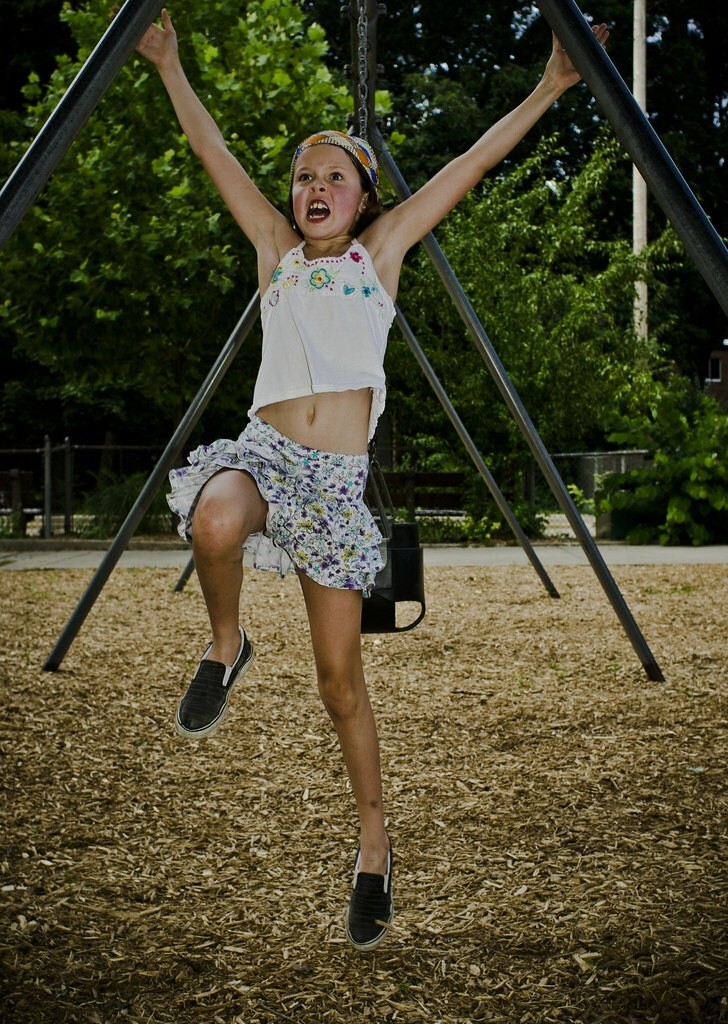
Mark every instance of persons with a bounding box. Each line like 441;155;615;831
118;8;608;947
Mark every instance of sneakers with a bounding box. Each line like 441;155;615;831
173;625;255;739
346;833;395;951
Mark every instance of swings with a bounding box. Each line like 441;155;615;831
358;0;426;634
